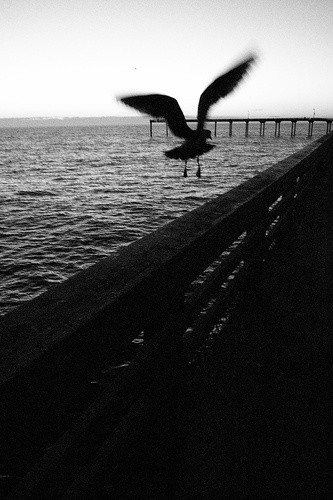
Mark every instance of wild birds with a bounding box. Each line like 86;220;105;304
119;57;255;179
